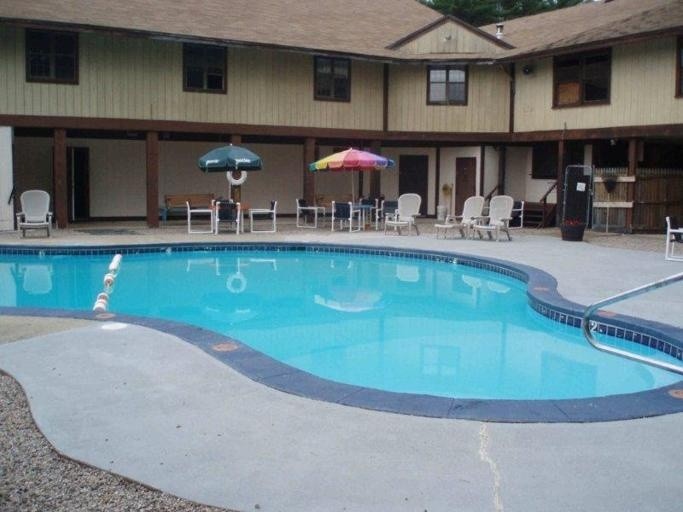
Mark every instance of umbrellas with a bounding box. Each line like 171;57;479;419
196;144;262;204
313;261;392;314
197;289;266;328
308;147;398;208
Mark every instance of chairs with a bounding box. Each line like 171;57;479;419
15;189;52;237
664;214;683;261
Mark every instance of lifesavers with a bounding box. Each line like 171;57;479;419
225;273;248;294
226;170;248;186
